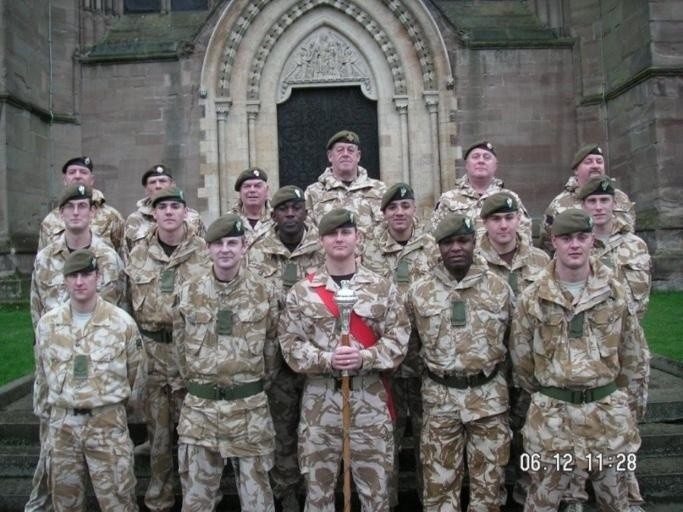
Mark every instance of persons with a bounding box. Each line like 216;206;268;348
24;156;149;512
277;209;411;512
358;183;444;511
473;192;551;508
226;167;327;511
304;130;389;268
405;214;516;511
510;209;650;511
125;164;215;511
538;143;636;261
431;141;534;252
554;175;651;511
171;213;278;512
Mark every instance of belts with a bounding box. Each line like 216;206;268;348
427;364;500;389
186;380;264;401
539;381;618;405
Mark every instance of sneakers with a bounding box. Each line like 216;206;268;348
282;489;299;512
629;505;645;512
564;501;584;512
134;440;149;456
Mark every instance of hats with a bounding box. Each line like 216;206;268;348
319;208;355;236
551;208;593;237
464;140;498;160
204;213;244;242
271;184;306;209
63;249;98;276
326;130;360;150
481;192;517;219
579;175;614;200
62;156;93;175
435;212;475;244
142;165;173;187
60;183;92;209
151;187;186;208
380;182;415;211
234;167;267;191
569;143;603;170
